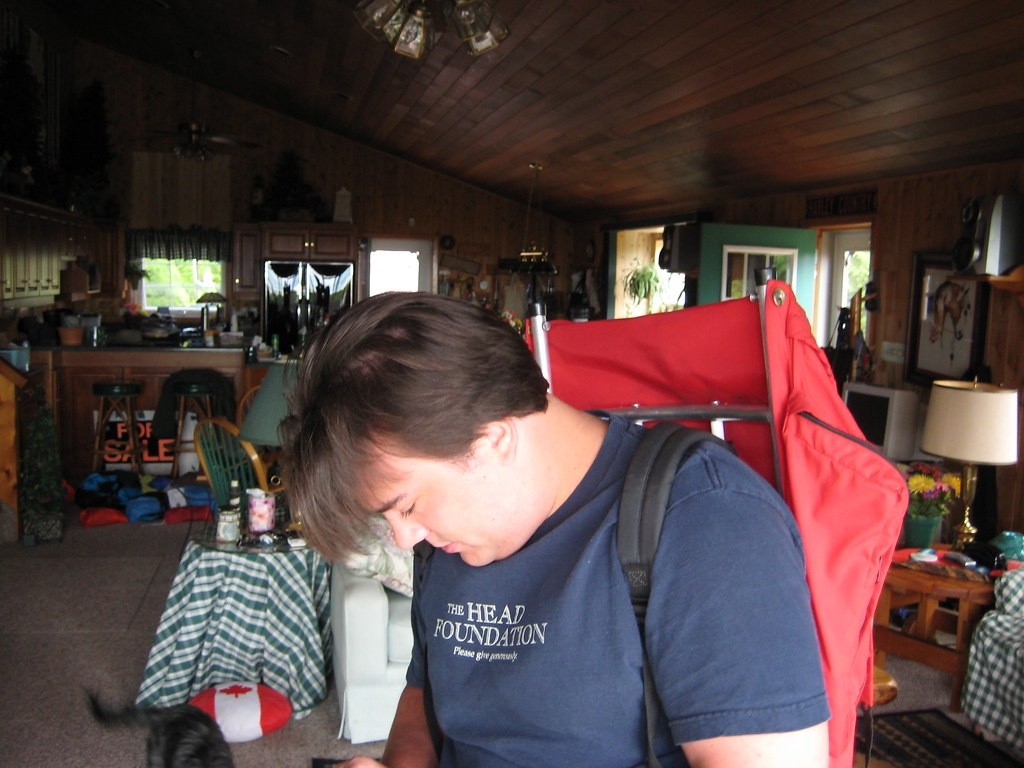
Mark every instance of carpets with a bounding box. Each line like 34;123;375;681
857;699;1024;768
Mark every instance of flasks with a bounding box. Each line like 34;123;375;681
201;307;208;334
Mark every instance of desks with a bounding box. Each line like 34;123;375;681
134;530;340;718
872;551;1011;712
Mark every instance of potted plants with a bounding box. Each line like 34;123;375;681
20;400;63;544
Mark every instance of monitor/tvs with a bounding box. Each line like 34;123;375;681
841;382;944;462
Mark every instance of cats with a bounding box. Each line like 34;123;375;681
80;685;234;768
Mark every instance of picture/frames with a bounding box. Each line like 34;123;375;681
905;249;997;383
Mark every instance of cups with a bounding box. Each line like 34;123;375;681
86;326;104;348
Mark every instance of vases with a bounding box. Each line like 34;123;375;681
904;512;943;547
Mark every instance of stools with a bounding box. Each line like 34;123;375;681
85;377;143;488
148;369;236;490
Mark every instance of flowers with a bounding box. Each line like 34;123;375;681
894;461;960;519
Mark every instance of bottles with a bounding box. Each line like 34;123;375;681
271;334;279;351
214;504;241;543
230;313;238;332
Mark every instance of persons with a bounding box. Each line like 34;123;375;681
282;292;831;768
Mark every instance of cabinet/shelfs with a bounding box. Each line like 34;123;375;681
49;342;247;493
229;221;260;301
261;222;359;259
0;191;124;300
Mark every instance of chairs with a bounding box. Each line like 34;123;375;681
192;419;293;519
527;281;910;767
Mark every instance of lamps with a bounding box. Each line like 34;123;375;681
196;291;226;328
353;0;510;58
511;159;560;277
237;366;314;525
169;143;216;165
928;379;1018;546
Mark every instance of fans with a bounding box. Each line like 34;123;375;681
144;42;244;148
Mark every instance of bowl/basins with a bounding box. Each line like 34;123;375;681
58;327;84;347
